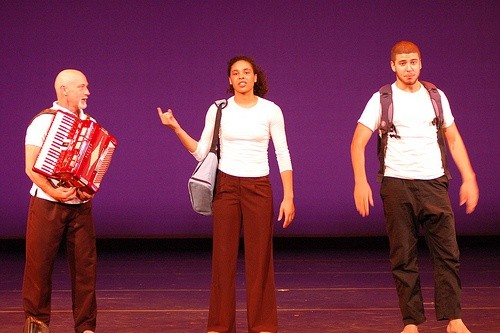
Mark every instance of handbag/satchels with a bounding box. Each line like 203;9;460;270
188;152;219;216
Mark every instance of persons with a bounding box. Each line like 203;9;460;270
350;41;479;333
156;55;296;333
24;68;97;333
